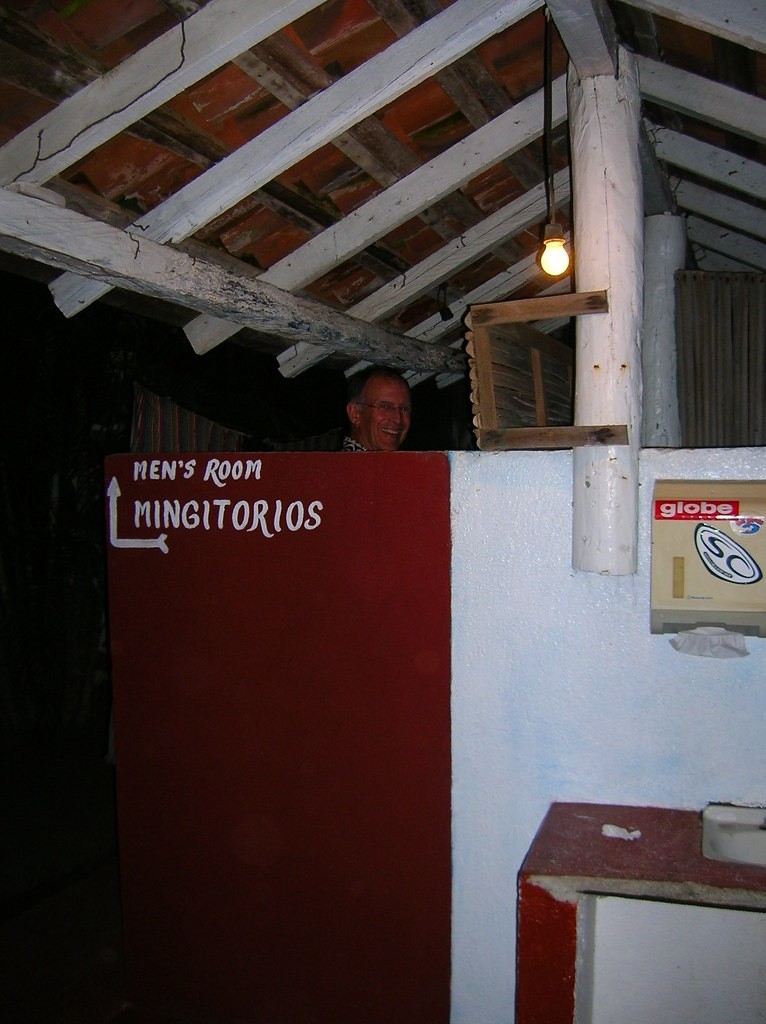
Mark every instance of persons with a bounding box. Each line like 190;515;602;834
340;362;412;453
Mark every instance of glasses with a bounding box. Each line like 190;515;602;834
356;401;411;414
